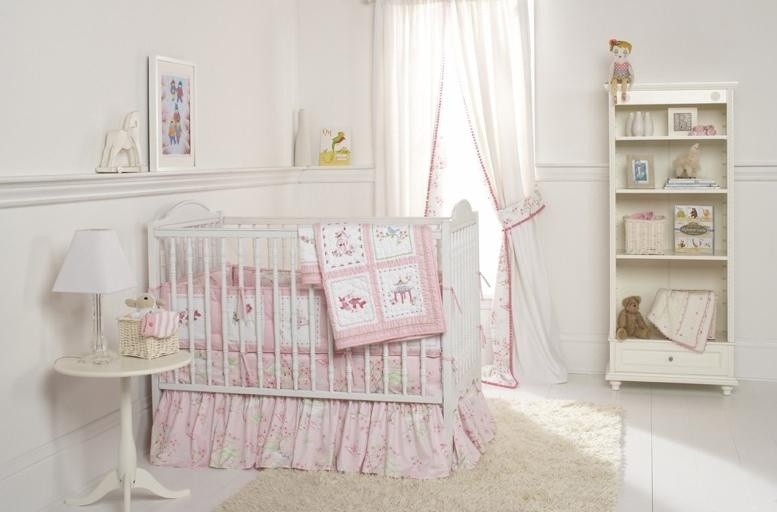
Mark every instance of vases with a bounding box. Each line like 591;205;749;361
625;111;654;136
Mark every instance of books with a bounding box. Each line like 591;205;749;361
673;203;715;256
318;126;352;166
663;176;720;190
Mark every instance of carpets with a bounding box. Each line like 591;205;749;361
211;397;624;512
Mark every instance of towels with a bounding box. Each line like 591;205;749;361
648;288;716;353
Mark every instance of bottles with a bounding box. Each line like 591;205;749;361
294;109;311;166
624;111;653;137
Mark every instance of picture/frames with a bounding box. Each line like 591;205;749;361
667;107;698;138
149;55;197;172
627;152;655;190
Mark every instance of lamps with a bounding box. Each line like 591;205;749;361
51;229;136;365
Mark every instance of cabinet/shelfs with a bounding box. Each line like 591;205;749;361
604;81;739;395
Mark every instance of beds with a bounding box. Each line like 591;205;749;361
147;199;497;480
